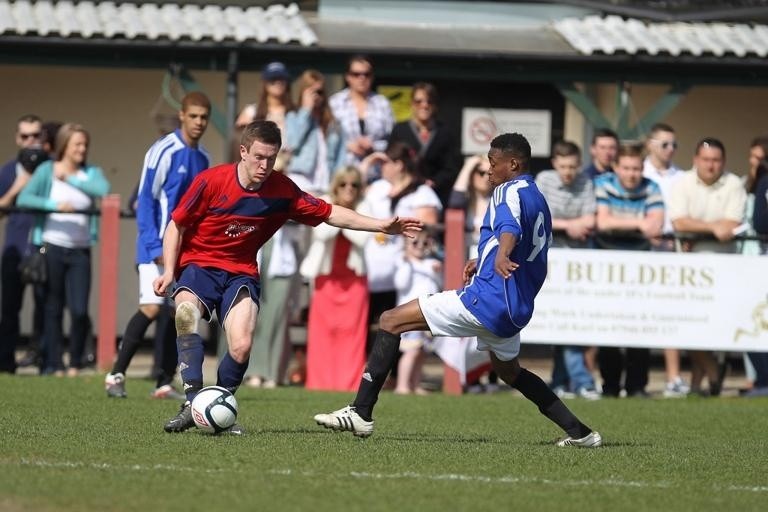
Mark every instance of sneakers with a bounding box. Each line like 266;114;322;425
165;400;194;433
555;432;600;451
151;389;185;402
310;409;374;438
101;374;122;403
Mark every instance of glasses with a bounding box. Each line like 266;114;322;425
408;241;430;247
350;73;372;80
475;170;492;178
410;103;435;106
316;89;326;97
19;132;40;143
655;141;681;152
337;180;363;192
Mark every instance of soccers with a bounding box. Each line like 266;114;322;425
190;386;239;433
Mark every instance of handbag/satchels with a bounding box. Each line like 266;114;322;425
17;243;45;295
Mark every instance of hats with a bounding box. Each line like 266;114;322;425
260;64;288;82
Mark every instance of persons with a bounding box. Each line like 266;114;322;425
326;56;394;186
583;129;620;247
393;226;442;396
154;119;422;430
17;124;110;375
733;137;764;387
593;145;664;398
670;135;751;395
448;155;498;392
391;82;463;207
283;71;342;197
747;156;764;386
105;89;212;401
314;132;603;448
536;142;600;399
0;116;51;375
639;123;692;395
246;149;318;386
290;161;366;386
236;61;293;131
358;146;441;387
